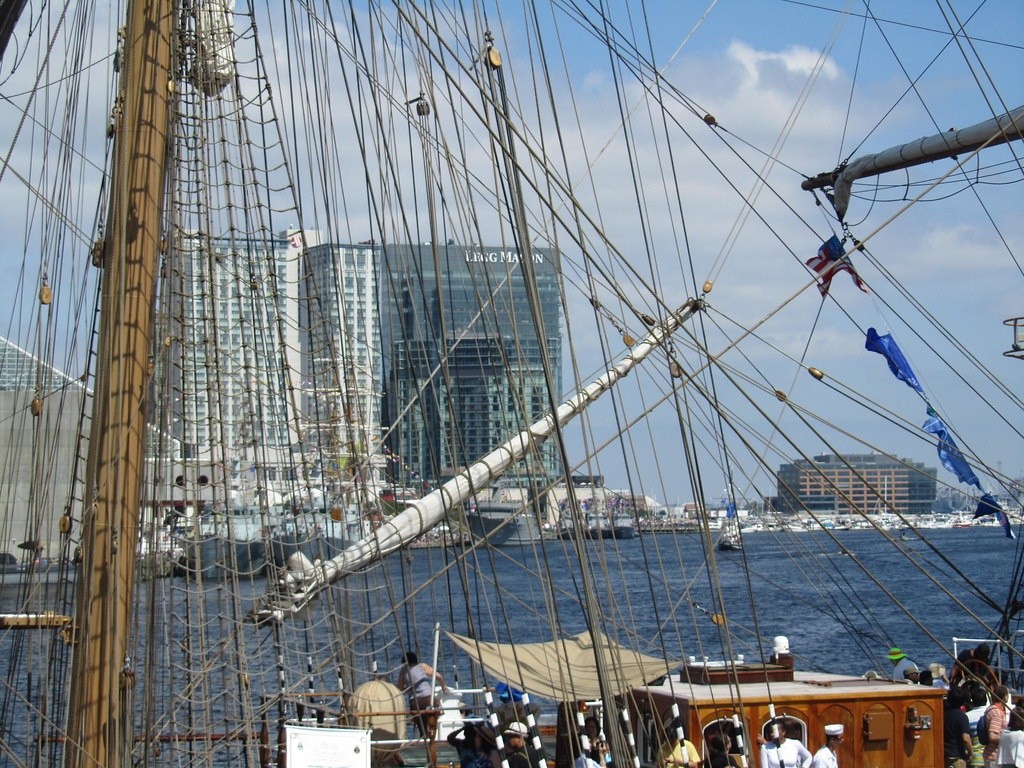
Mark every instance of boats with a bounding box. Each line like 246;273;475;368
754;523;782;532
952;520;972;528
973;518;1001;527
175;489;283;582
828;524;849;530
916;521;953;529
556;498;635;540
466;499;534;546
404;533;461;549
252;487;372;571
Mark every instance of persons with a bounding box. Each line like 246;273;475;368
447;722;494;768
704;732;741;768
460;706;494;732
505;721;540;768
583;717;605;764
982;685;1024;768
904;643;1007;713
397;651;448;738
482;681;534;703
943;688;974;768
759;719;812;768
570;732;607;768
884;648;918;681
654;719;703;768
812;723;844;768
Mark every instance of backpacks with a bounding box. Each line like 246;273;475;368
977;704;997;745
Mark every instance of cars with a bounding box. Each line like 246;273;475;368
0;553;23;573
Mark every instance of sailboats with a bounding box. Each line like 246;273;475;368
711;467;744;550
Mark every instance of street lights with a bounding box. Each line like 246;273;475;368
13;539;18;557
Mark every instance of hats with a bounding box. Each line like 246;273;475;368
504;722;528;737
886;648;908;659
929;663;945;678
825;724;843;735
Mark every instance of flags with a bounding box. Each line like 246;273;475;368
806;234;868;296
